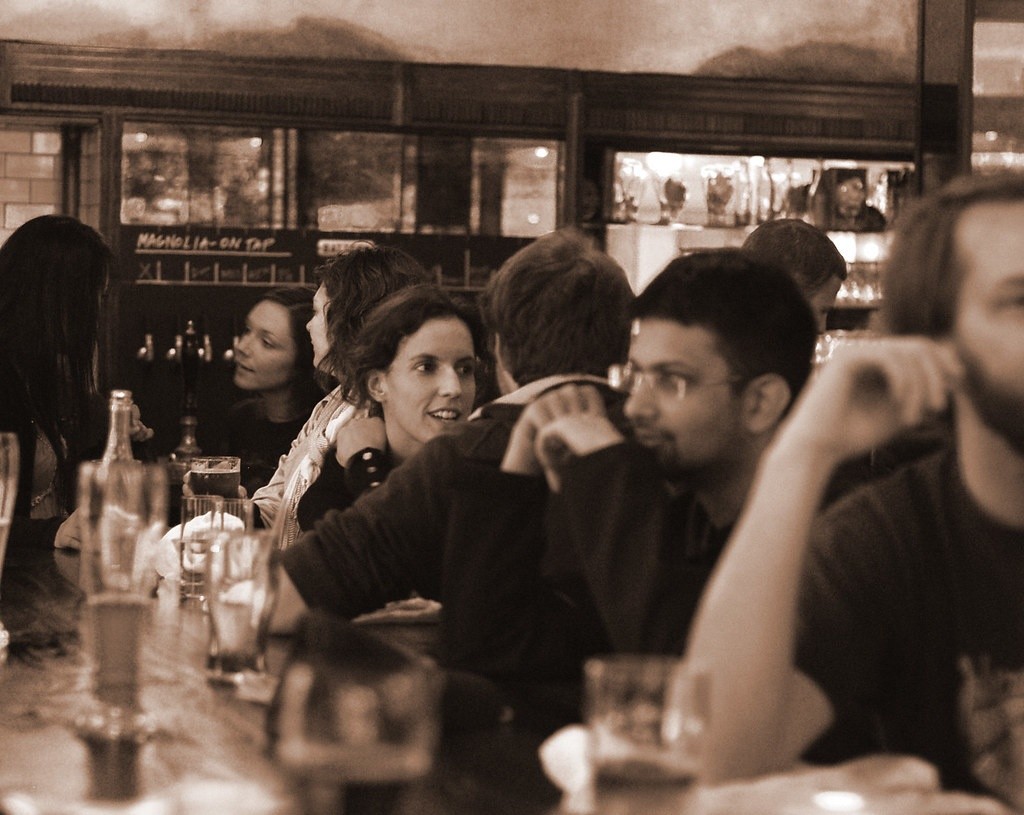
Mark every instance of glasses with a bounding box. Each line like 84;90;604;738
607;363;742;401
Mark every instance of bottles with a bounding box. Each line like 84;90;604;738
77;390;169;596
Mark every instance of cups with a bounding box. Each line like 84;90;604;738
190;457;242;498
179;495;253;600
205;530;280;687
584;653;693;815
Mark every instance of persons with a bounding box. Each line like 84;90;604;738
672;168;1024;815
298;285;483;534
741;219;849;336
0;214;154;550
250;245;429;550
441;246;816;682
275;229;633;621
219;288;317;498
